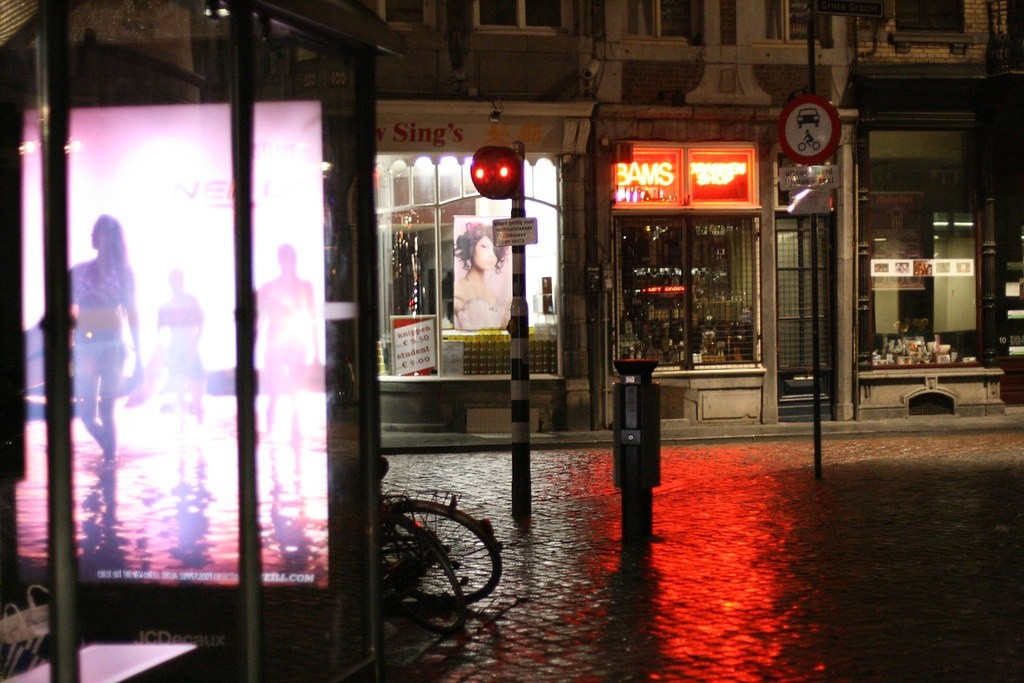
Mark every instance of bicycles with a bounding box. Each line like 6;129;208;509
380;457;502;631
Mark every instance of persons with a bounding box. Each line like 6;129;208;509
69;214;143;464
455;221;508;331
159;269;207;429
255;243;320;450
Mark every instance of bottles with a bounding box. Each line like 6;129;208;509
618;286;760;368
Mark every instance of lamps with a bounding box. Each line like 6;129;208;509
489;95;505;121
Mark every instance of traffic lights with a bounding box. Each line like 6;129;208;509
470;153;520;200
